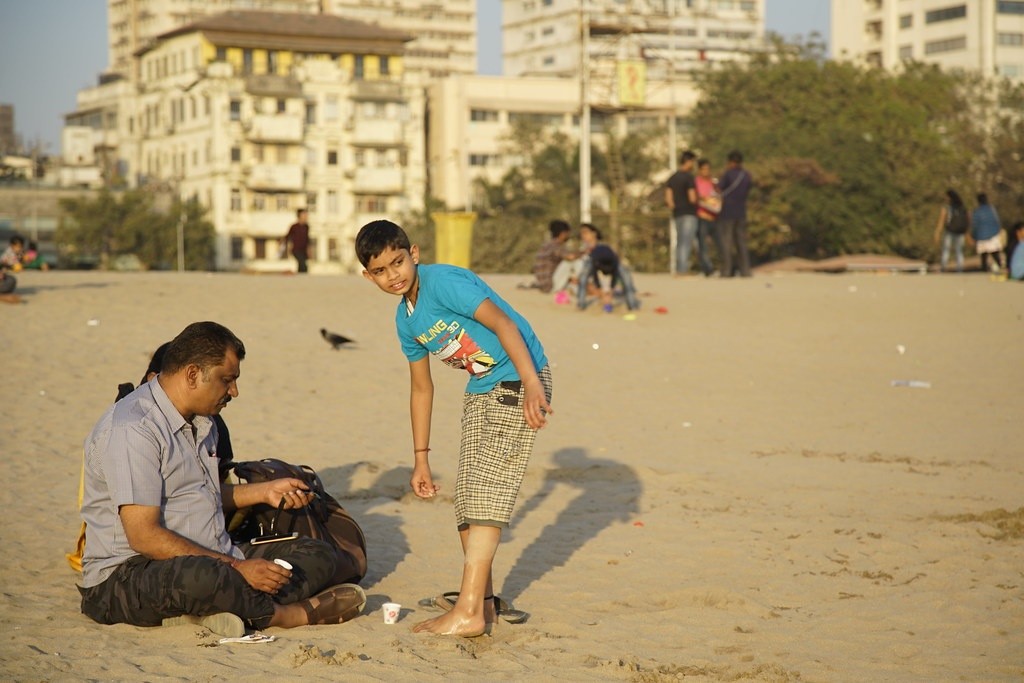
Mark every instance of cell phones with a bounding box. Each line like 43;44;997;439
251;532;298;545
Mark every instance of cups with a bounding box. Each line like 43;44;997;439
274;559;293;571
382;603;402;624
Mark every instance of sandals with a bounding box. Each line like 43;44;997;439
298;582;367;626
179;612;245;639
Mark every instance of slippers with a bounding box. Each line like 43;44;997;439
418;592;460;612
494;595;527;624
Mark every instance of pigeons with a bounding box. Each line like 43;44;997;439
320;328;355;348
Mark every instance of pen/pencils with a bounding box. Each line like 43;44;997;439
207;451;213;458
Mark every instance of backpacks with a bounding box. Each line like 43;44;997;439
945;208;967;234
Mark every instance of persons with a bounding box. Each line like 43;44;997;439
284;209;313;273
534;223;639;312
934;189;1024;279
0;238;50;304
665;149;754;277
65;322;365;638
355;220;554;638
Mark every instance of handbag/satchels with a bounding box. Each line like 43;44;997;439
235;456;368;586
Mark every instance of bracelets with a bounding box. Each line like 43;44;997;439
413;448;431;452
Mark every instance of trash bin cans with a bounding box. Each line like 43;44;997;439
430;212;478;269
